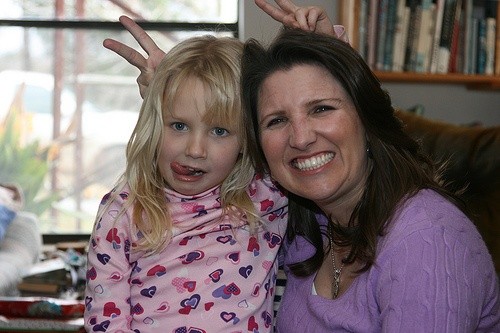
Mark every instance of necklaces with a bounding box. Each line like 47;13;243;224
329;223;348;299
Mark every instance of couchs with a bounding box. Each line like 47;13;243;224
394;110;500;265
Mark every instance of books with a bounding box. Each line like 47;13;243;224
359;0;500;75
17;257;68;299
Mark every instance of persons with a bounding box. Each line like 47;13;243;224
103;15;500;333
84;1;349;333
